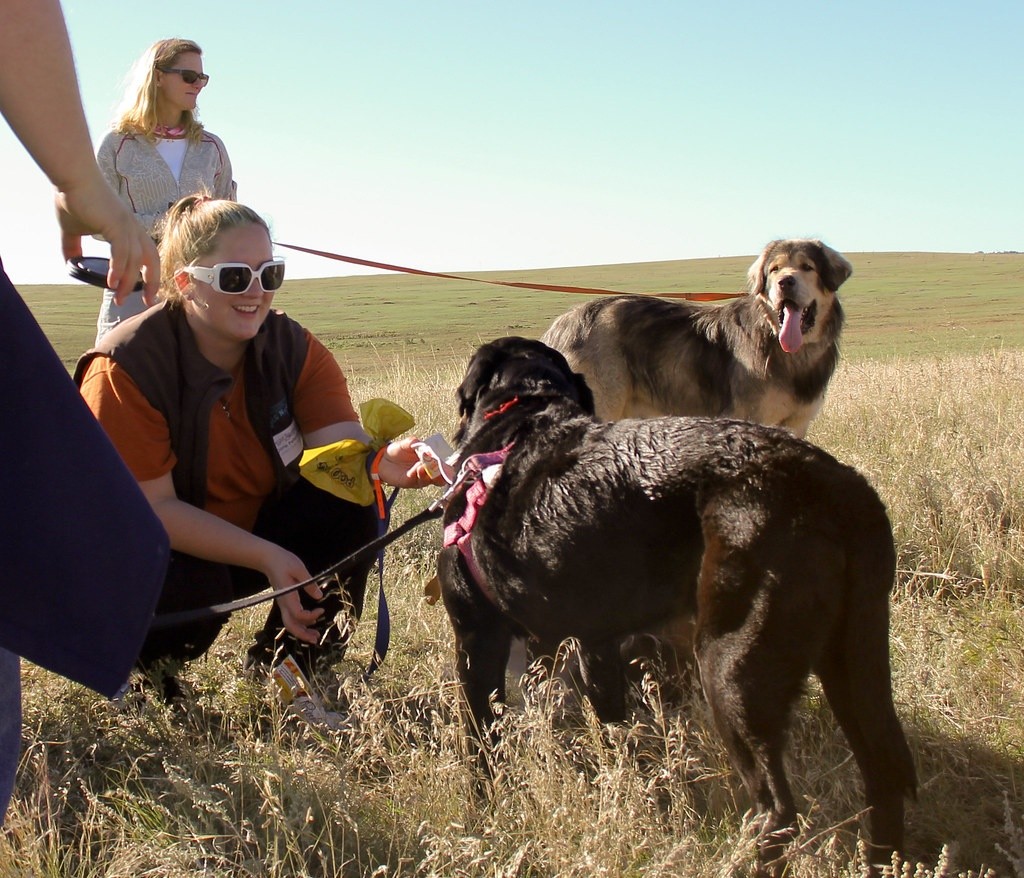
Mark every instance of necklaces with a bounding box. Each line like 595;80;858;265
221;365;242;410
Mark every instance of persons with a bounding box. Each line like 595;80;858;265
71;193;444;727
96;37;237;346
0;0;174;813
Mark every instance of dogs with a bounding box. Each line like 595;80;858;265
540;239;855;440
432;336;915;871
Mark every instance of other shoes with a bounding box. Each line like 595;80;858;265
296;662;354;733
130;673;197;713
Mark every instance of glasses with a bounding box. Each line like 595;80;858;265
158;68;210;88
183;260;285;294
